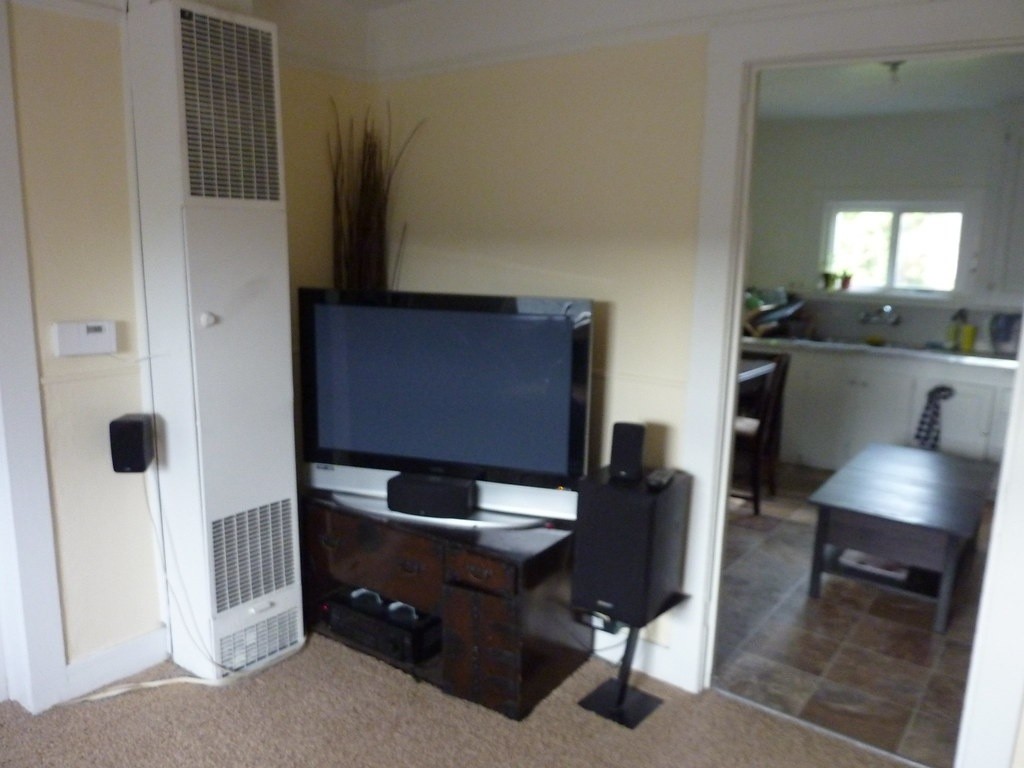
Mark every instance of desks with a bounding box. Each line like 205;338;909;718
738;358;776;386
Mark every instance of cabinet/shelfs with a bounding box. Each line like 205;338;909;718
907;374;1014;504
778;359;914;473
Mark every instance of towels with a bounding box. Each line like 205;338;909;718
915;386;955;450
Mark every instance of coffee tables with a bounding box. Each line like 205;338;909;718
806;440;1000;634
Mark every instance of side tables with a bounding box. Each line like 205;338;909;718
297;485;596;723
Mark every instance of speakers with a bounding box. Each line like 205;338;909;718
109;413;156;474
610;422;645;479
569;465;693;625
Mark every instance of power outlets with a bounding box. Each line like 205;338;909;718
49;322;116;359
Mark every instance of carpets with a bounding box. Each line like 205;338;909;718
1;631;933;768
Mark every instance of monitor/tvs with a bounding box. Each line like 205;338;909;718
297;285;596;493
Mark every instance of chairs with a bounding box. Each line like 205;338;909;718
728;352;789;515
